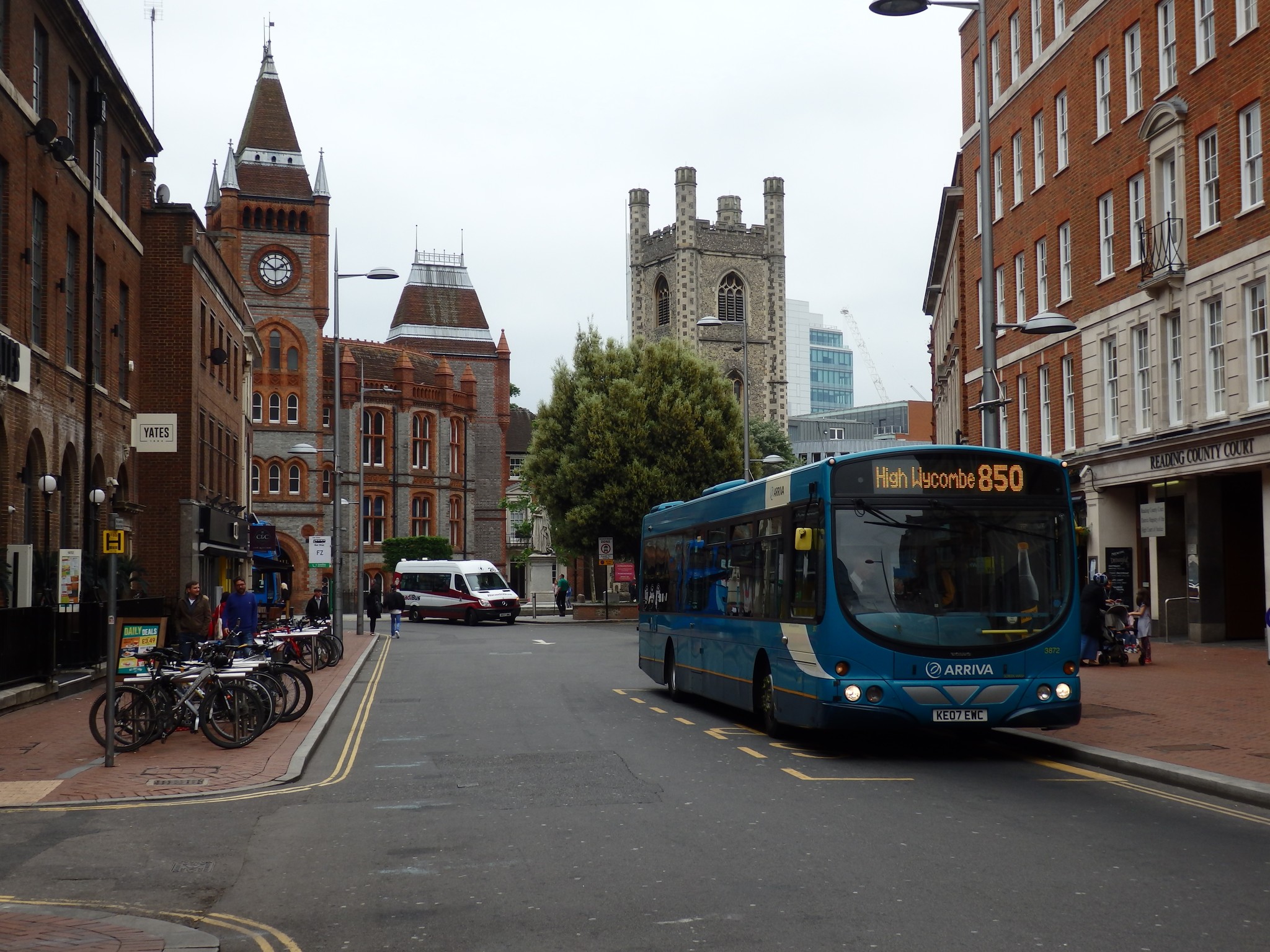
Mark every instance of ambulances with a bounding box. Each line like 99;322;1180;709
392;554;523;628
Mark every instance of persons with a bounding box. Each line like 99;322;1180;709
1081;571;1153;666
407;576;448;591
553;574;572;617
224;576;258;659
179;580;210;644
367;587;384;636
726;600;745;613
371;579;381;600
280;583;290;610
844;550;887;601
893;578;905;602
212;591;232;640
383;583;405;639
305;588;331;624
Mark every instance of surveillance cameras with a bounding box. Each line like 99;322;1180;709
1079;465;1091;478
249;512;259;525
110;479;119;487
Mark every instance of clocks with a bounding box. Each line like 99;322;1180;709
249;244;301;295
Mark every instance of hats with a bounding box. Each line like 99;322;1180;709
202;595;209;601
390;584;397;586
314;588;322;592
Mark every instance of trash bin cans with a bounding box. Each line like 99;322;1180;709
604;590;620;603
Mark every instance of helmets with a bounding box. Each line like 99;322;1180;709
1093;573;1108;586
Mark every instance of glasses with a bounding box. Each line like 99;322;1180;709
236;584;246;587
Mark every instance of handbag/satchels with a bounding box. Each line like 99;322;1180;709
369;593;382;614
214;604;223;640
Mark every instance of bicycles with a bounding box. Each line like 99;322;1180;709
86;614;346;750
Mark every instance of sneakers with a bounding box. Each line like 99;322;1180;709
392;636;395;638
395;630;400;639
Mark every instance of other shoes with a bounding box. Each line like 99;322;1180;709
559;606;572;616
1079;645;1152;667
370;632;374;635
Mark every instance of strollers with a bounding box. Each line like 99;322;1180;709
1096;604;1139;665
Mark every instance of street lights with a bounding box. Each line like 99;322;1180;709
860;0;1077;451
89;489;107;600
698;315;788;483
39;474;61;611
289;229;401;659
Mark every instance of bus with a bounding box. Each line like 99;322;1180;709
251;521;283;613
629;439;1087;741
